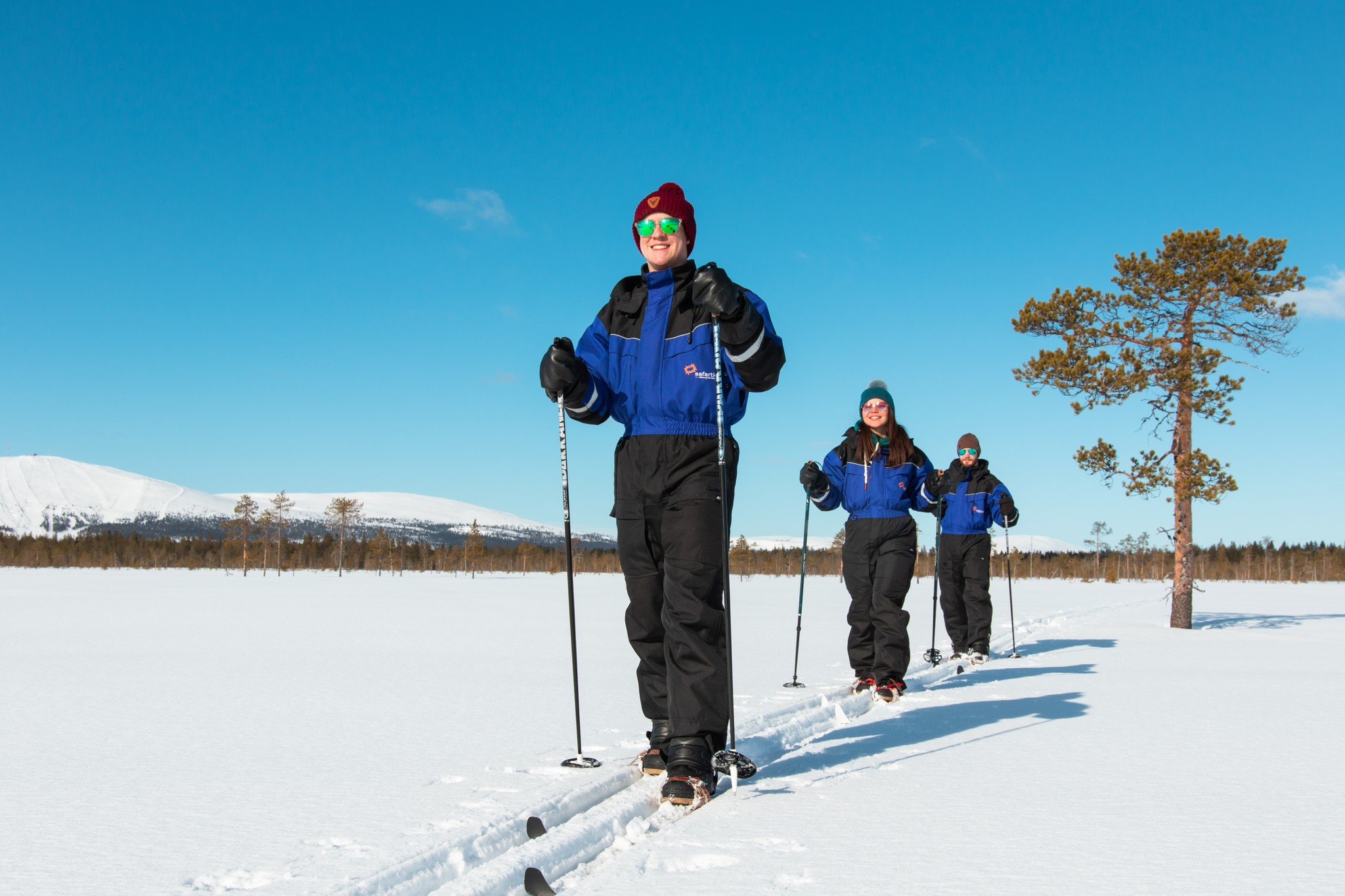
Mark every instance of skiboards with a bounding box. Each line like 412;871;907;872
524;788;729;896
933;661;964;675
821;695;877;724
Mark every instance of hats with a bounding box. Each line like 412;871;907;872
957;433;980;458
632;182;696;258
859;379;895;420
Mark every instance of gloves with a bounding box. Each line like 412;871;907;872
926;469;953;497
693;267;760;344
800;462;825;494
997;492;1014;516
931;500;948;518
540;337;590;407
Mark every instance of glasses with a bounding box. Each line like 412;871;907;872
861;402;888;412
958;448;977;456
634;217;682;238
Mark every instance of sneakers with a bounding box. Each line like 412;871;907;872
874;678;904;701
856;678;876;694
971;644;989;665
949;650;961;660
660;736;717;804
643;719;670;775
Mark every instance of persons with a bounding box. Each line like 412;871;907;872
931;432;1019;665
798;379;951;703
540;181;785;806
